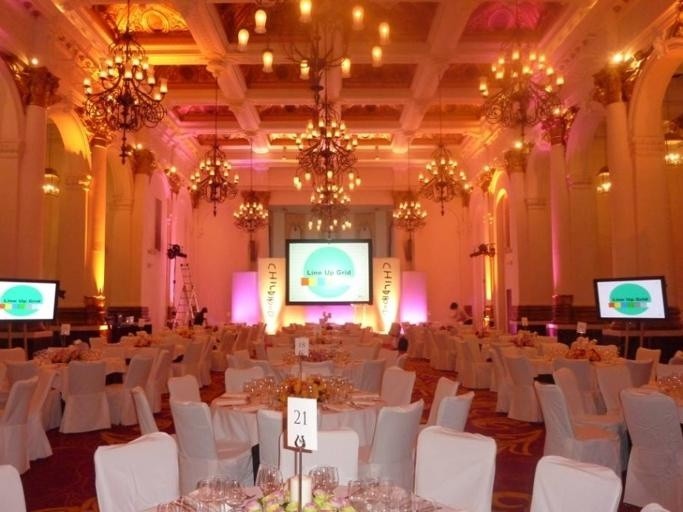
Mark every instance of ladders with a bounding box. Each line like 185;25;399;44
173;264;199;330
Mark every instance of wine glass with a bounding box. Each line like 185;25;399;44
311;466;436;512
156;463;283;512
241;375;353;411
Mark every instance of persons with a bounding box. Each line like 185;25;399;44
193;307;207;325
448;302;472;325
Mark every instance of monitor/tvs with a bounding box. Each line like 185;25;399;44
286;238;372;303
592;275;668;321
0;277;60;321
